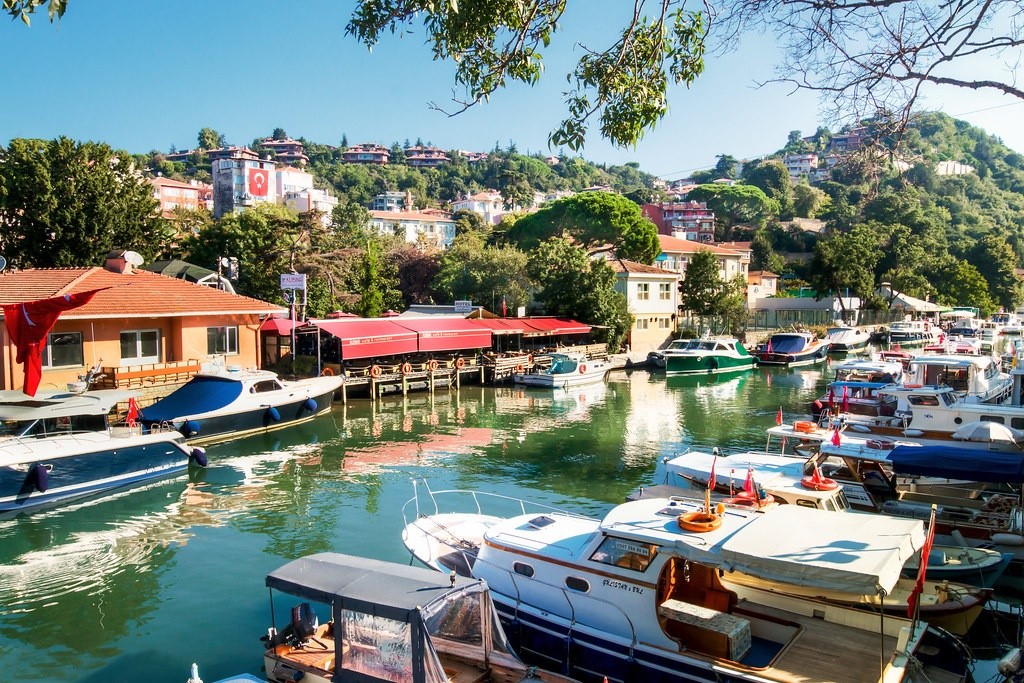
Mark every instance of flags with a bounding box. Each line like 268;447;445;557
710;459;719;490
843;385;850;411
503;297;507;316
906;510;936;618
125;397;139;427
0;284;116;397
812;466;824;484
829;388;836;409
249;168;268;196
766;339;772;357
743;466;754;493
775;409;781;424
830;427;840;447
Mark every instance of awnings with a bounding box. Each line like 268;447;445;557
309;316;594;361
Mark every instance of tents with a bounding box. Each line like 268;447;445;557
940;310;975;327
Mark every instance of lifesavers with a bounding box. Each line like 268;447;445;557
402;363;412;375
516;364;523;373
370;366;381;378
579;364;587;374
801;476;839;490
528;353;533;363
678;511;723;533
430;360;438;371
323;368;334;376
866;439;895;450
736;490;775;505
904;384;923;388
792;421;817;432
456;357;465;368
720;497;759;511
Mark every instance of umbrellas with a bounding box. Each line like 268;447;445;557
957;419;1024;451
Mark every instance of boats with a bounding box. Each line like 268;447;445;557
400;470;978;683
514;351;615;389
259;551;580;683
623;312;1024;658
0;388;208;523
111;349;348;450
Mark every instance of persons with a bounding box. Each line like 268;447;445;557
940;333;945;344
758;483;766;499
989;314;1005;326
925;317;929;332
876;373;898;399
